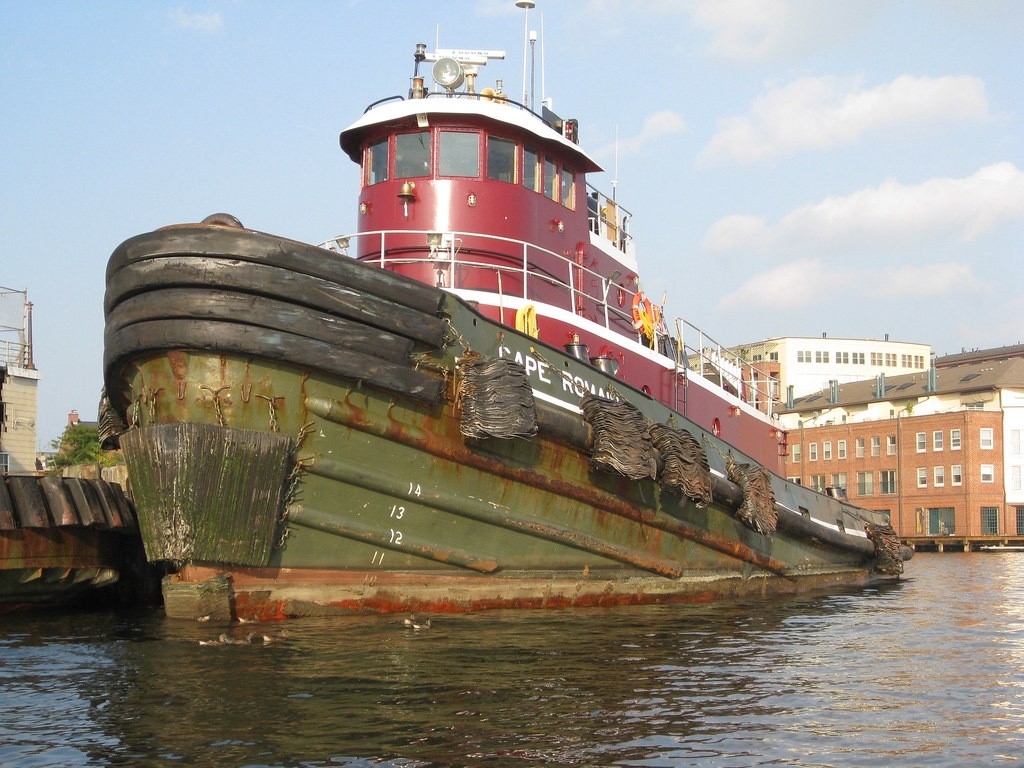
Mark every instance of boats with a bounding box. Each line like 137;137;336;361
104;0;914;624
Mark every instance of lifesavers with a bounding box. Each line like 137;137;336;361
632;292;652;335
